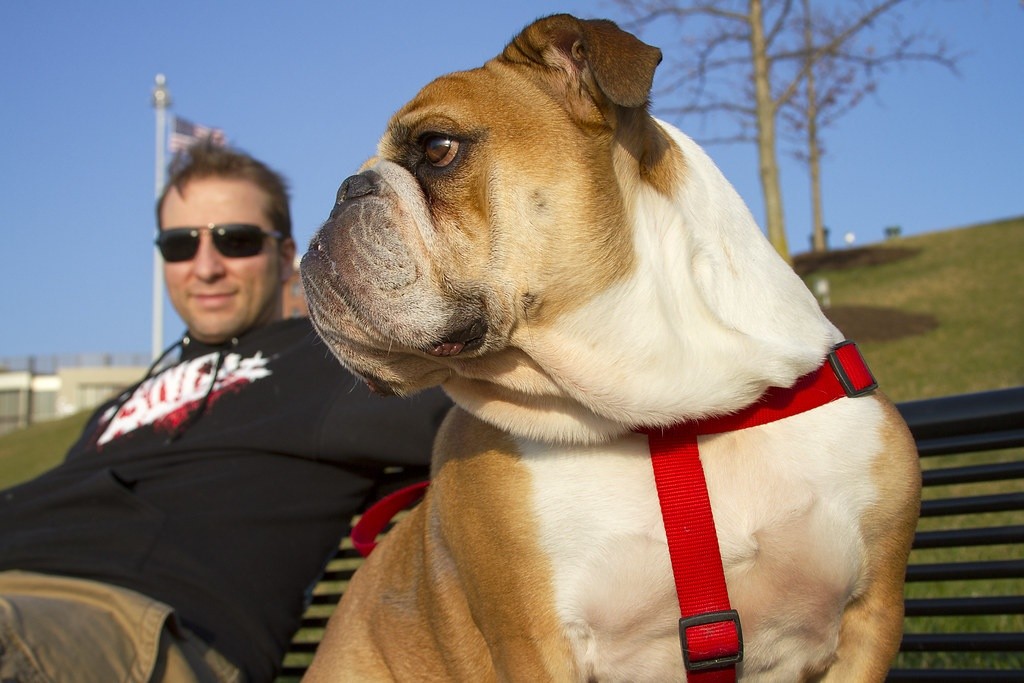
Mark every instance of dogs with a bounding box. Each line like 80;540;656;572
298;10;925;683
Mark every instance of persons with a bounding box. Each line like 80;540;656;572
1;131;455;683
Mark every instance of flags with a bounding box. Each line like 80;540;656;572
169;118;226;153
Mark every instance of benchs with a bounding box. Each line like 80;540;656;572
274;389;1024;683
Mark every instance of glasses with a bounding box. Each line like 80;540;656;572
155;223;286;262
